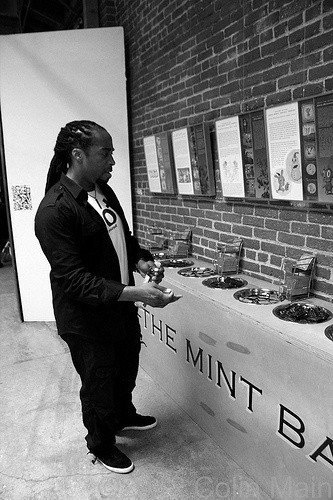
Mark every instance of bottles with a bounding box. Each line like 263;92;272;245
135;261;160;308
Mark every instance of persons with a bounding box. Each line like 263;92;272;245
33;119;182;474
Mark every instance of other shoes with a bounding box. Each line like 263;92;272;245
120;413;158;432
87;444;134;473
